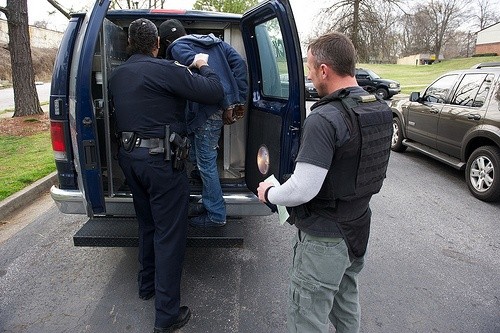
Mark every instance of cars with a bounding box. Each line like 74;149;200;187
276;73;320;102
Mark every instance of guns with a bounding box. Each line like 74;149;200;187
170;132;189;171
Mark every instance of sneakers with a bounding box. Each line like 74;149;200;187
189;215;226;227
190;169;201;180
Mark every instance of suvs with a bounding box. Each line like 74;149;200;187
387;62;500;203
354;67;402;100
46;0;309;249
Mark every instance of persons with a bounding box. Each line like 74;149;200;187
258;31;394;333
158;19;248;227
109;19;225;333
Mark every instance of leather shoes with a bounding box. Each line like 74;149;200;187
140;291;154;299
154;306;191;333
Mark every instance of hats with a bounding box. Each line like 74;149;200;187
159;19;185;39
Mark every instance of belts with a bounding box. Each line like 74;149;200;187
135;139;166;151
